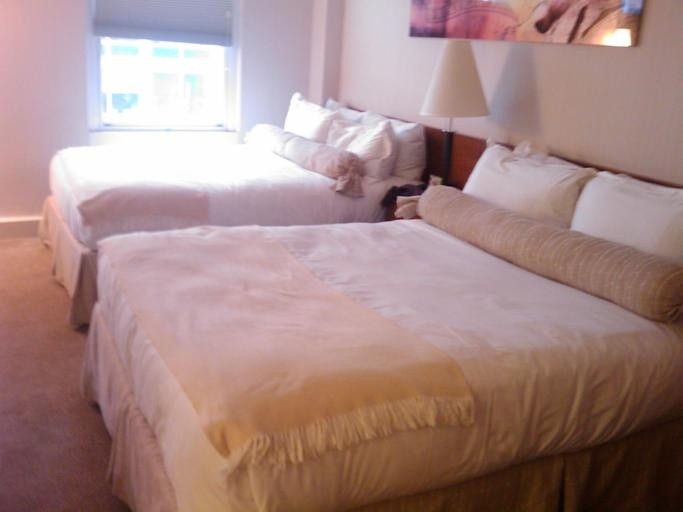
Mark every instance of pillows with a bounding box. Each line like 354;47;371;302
394;173;682;324
460;145;594;229
570;168;682;267
242;92;425;198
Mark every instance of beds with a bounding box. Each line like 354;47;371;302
82;151;683;511
38;103;453;317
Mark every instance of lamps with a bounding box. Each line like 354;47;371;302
422;38;488;180
